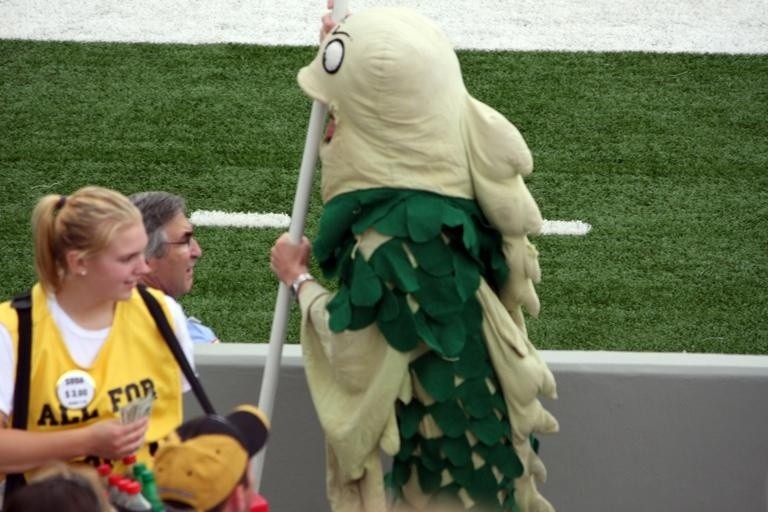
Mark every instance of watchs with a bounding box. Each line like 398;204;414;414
289;272;315;305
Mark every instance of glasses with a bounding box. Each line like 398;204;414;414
153;231;192;253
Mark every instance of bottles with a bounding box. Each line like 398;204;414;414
96;455;165;511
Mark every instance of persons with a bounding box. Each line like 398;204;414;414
131;194;218;346
267;0;567;512
0;184;194;511
0;474;103;511
